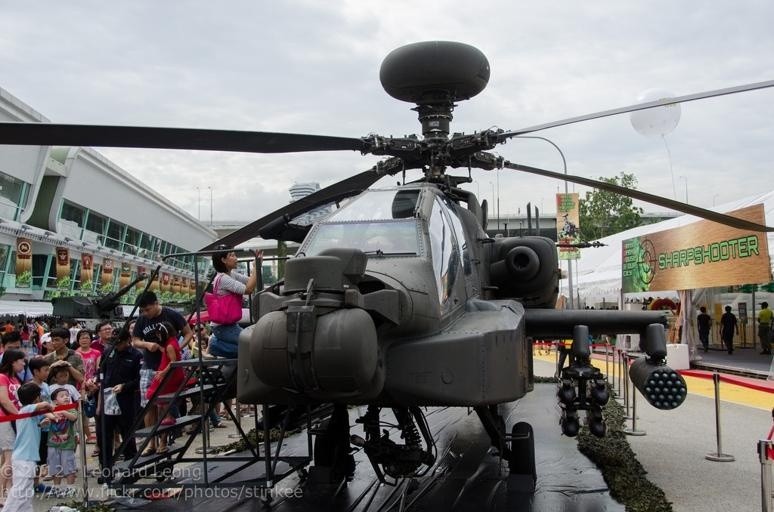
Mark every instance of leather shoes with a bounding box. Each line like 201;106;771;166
97;472;115;485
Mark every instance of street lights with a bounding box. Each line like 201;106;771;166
710;193;720;207
473;180;480;201
206;184;213;224
488;180;495;216
679;174;690;204
193;185;200;221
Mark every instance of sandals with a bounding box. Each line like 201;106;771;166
160;416;178;425
140;444;171;457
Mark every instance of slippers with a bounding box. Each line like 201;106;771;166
213;421;227;428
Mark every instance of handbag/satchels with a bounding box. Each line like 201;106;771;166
204;291;243;324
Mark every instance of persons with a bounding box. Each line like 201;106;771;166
94;328;139;483
27;355;54;492
0;348;24;506
719;305;738;354
203;244;262;358
696;306;711;351
0;309;256;460
2;382;54;512
131;290;193;455
757;302;774;355
42;388;79;497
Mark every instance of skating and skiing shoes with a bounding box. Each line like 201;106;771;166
33;482;79;499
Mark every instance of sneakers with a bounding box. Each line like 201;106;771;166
86;435;97;444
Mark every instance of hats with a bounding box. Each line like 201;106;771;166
106;328;130;346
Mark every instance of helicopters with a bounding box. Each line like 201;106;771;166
0;40;773;512
